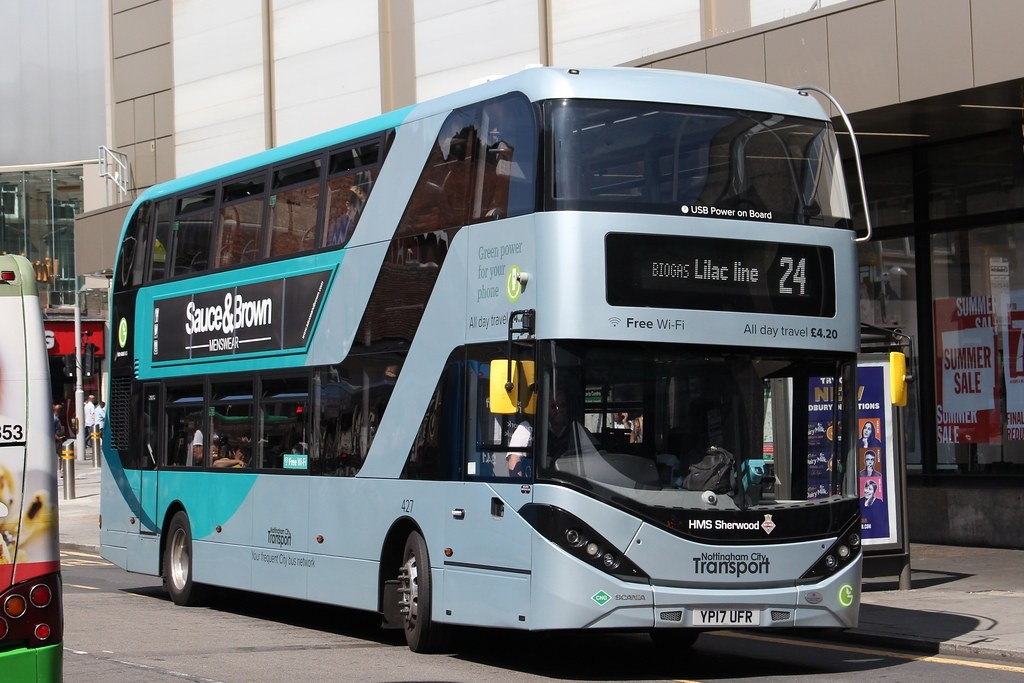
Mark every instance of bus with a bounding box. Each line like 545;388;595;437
0;254;63;683
100;72;915;653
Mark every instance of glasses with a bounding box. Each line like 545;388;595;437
550;403;567;409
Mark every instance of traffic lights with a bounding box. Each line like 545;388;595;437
84;343;101;377
61;354;76;378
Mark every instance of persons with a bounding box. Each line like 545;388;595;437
505;386;598;477
858;450;882;477
190;409;254;470
48;395;105;477
610;398;643;445
860;481;882;507
857;421;882;447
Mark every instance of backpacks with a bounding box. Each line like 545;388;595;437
683;446;745;509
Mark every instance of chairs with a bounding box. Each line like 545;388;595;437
151;224;316;281
